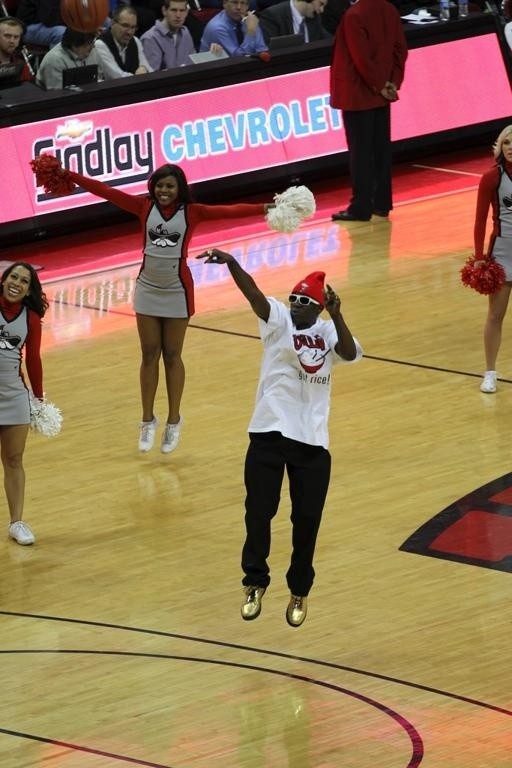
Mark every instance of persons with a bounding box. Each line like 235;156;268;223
329;1;407;221
49;158;277;454
193;247;365;627
0;261;50;545
471;117;512;398
2;0;512;105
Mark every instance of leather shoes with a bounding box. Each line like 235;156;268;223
331;211;370;220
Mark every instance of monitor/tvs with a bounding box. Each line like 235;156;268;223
62;64;98;90
269;33;305;51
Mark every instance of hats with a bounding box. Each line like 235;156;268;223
292;271;326;310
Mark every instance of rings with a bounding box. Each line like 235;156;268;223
209;253;212;256
207;250;213;254
335;295;339;300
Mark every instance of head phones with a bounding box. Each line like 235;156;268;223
0;16;26;51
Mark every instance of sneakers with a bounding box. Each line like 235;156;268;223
8;520;35;545
138;414;183;453
286;592;308;627
480;371;496;393
241;585;265;621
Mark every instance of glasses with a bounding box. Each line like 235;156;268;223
113;20;138;33
287;293;320;306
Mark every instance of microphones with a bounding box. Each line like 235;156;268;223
15;45;21;50
314;11;321;16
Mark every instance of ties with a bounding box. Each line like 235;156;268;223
234;21;245;46
299;17;306;43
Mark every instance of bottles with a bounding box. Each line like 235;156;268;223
439;0;450;21
458;1;469;16
